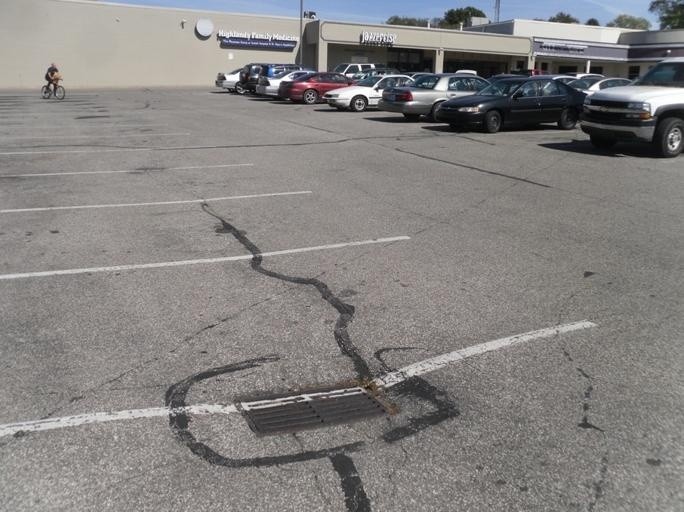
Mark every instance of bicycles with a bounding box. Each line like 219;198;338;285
41;78;65;100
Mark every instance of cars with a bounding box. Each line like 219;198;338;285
580;57;683;157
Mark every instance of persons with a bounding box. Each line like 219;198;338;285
45;63;59;96
